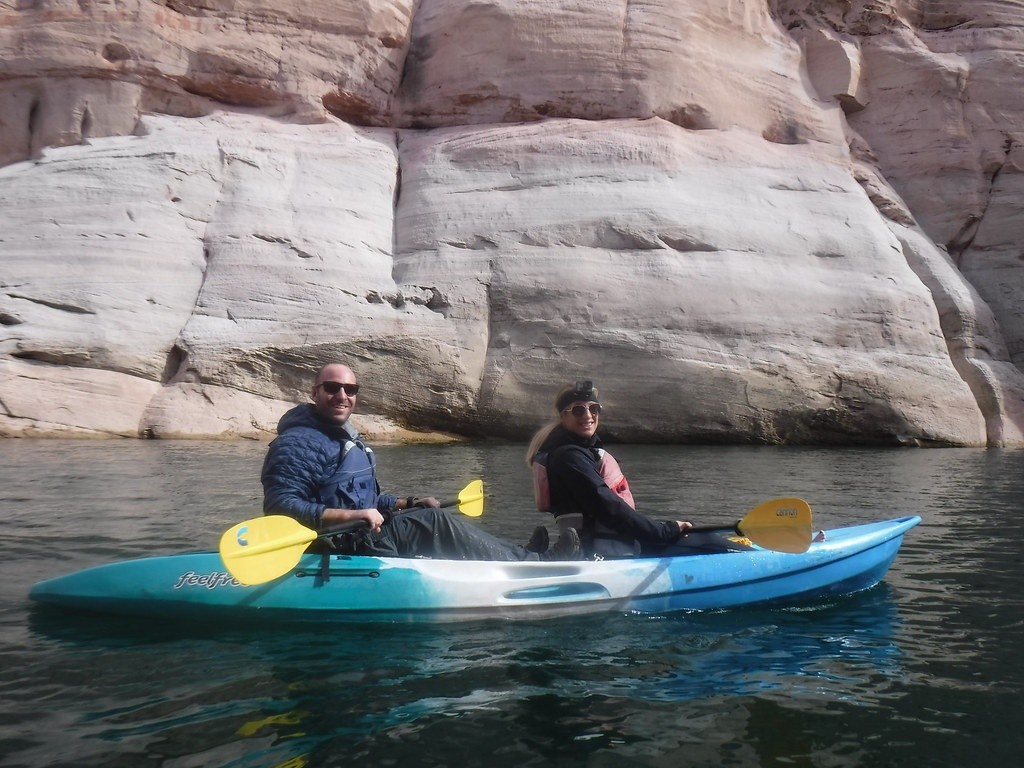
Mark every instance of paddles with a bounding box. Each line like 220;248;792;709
218;479;484;586
680;497;813;555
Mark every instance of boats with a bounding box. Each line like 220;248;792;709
26;513;923;625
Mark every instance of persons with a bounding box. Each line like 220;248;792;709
260;361;581;561
527;381;826;562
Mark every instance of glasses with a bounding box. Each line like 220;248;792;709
316;381;360;397
564;404;602;417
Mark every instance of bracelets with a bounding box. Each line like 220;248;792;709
406;497;419;509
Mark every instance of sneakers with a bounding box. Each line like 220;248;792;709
544;526;579;561
525;525;549;554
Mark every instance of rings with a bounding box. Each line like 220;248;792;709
376;522;380;525
371;521;374;524
